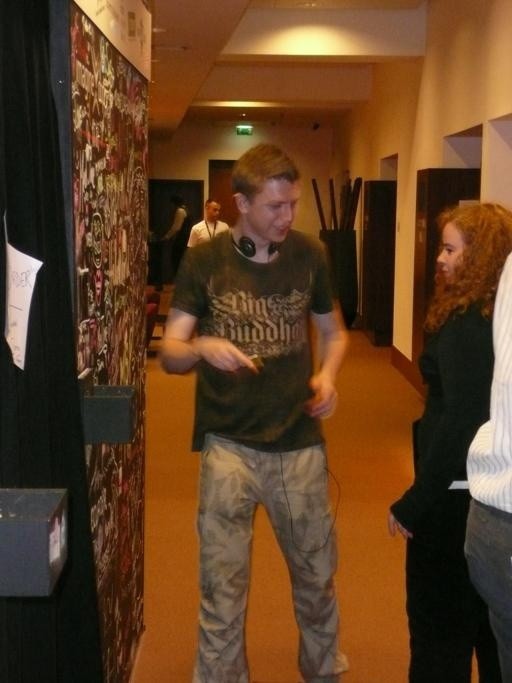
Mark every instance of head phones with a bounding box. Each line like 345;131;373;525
230;232;278;257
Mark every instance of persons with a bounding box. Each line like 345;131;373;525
463;249;512;682
385;199;512;681
157;141;348;683
186;197;230;247
153;192;193;272
